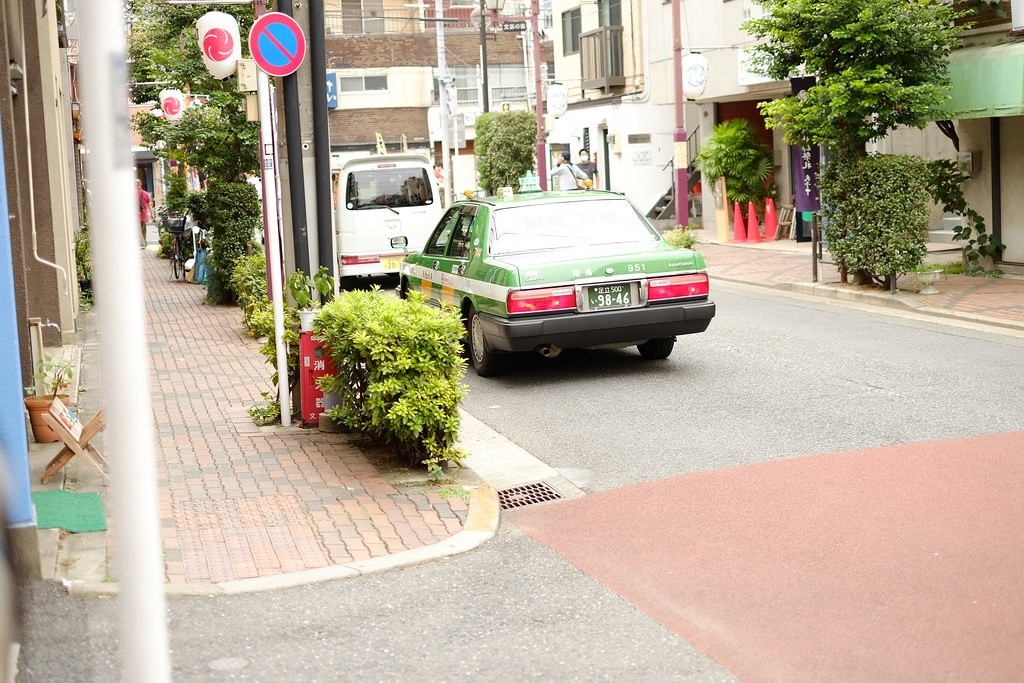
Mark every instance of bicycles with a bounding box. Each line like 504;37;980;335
160;209;186;282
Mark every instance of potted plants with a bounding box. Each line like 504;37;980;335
914;264;947;295
56;0;65;33
284;266;334;332
25;352;73;444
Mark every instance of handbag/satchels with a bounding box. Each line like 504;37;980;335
139;192;143;210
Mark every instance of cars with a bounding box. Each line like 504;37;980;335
390;169;716;376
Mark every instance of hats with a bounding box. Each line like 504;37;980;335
562;151;569;158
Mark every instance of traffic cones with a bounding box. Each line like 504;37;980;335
746;200;764;243
732;202;747;243
761;198;777;239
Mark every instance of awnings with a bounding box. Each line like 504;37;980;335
926;41;1024;122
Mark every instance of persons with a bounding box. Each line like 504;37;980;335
434;163;444;183
575;149;600;189
550;152;587;192
135;179;155;249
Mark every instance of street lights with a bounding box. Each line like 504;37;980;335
480;0;506;108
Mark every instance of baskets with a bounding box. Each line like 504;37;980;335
163;216;186;231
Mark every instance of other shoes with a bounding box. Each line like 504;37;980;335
141;246;146;249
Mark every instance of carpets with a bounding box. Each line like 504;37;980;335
32;490;107;531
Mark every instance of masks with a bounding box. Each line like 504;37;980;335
581;155;588;161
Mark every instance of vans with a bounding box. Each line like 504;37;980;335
335;153;442;280
